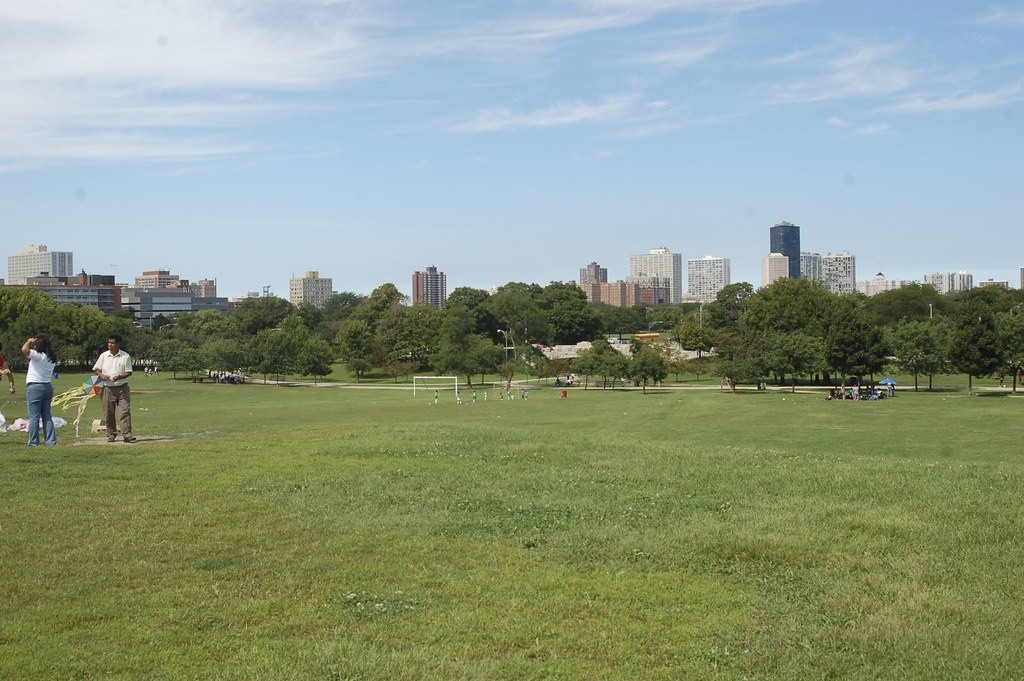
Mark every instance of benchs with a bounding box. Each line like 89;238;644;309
555;381;581;387
595;381;624;387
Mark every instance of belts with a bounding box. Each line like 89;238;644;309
27;383;50;386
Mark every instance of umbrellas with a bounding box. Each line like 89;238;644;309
879;378;896;384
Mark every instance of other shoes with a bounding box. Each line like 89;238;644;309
108;436;115;442
124;435;136;442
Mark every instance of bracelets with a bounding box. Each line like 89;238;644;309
119;374;121;379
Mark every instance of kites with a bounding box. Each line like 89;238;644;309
51;375;104;438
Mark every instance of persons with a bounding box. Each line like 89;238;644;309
21;333;58;446
144;366;158;376
554;372;574;387
0;342;15;394
826;382;895;400
434;387;528;403
193;371;245;384
92;333;136;442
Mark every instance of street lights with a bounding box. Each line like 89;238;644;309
497;329;508;377
649;320;663;334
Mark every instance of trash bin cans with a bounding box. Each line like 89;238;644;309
633;379;639;387
850;376;858;386
760;383;766;391
561;391;567;397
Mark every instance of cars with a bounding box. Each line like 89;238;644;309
607;337;630;344
